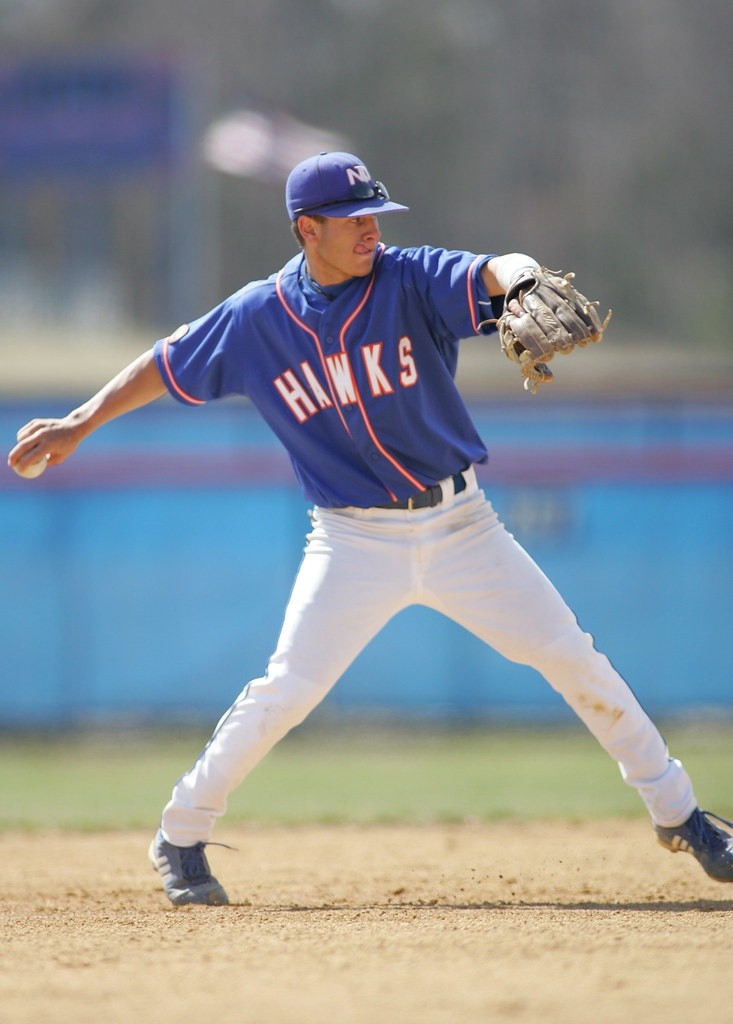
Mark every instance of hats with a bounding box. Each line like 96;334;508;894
286;152;410;219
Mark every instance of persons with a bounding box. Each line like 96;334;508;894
6;151;733;882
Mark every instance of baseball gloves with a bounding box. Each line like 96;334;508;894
476;266;614;396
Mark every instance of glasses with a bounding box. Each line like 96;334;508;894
293;181;389;214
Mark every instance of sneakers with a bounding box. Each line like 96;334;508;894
652;805;733;883
148;828;238;906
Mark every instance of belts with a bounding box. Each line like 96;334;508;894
376;474;466;512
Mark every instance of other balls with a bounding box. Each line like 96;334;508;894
12;454;48;479
